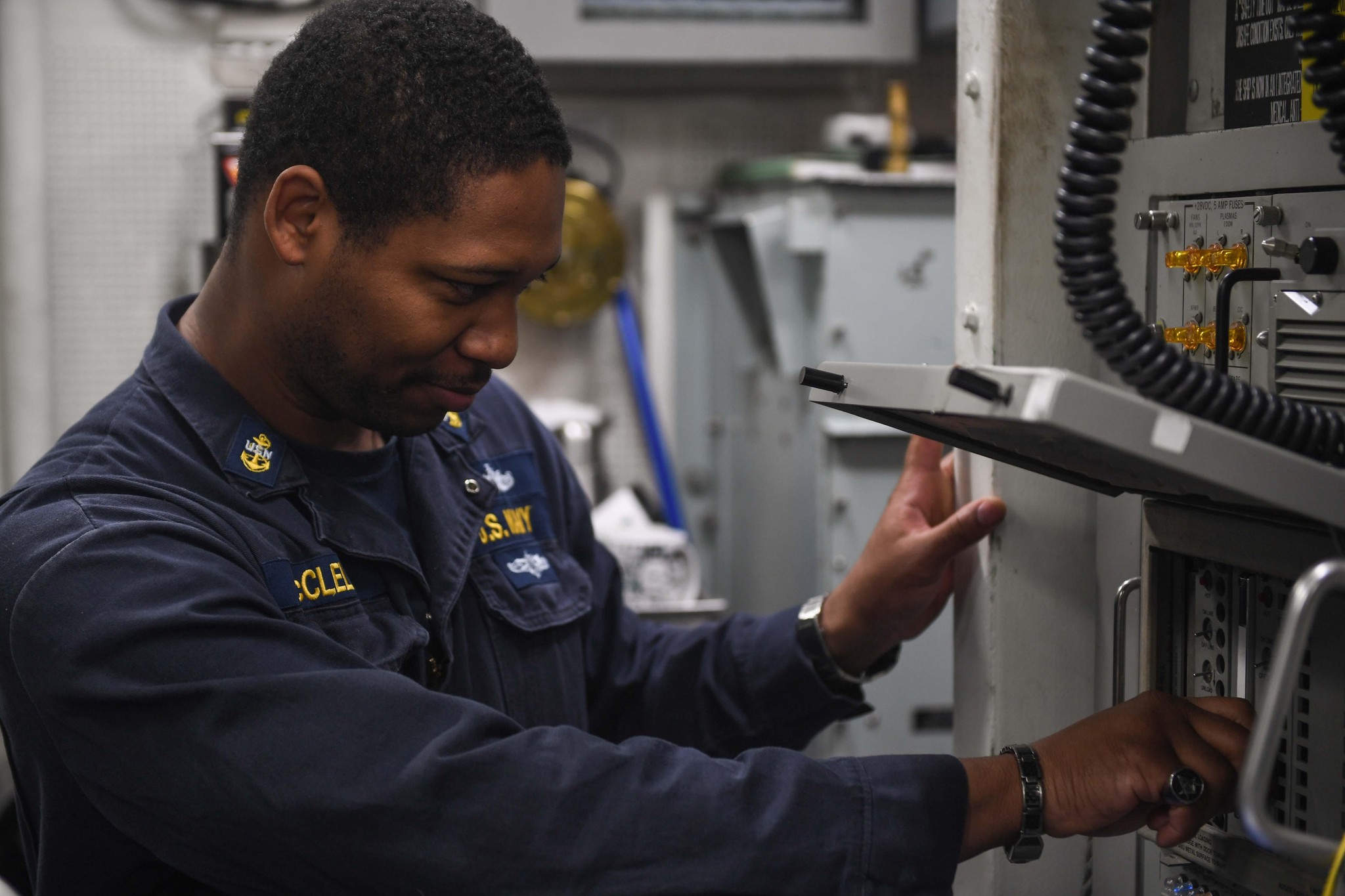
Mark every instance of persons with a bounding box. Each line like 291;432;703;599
0;0;1252;896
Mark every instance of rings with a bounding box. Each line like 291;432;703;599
1165;769;1208;805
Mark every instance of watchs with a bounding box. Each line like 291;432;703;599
795;594;900;685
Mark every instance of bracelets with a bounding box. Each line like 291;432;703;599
998;744;1045;864
